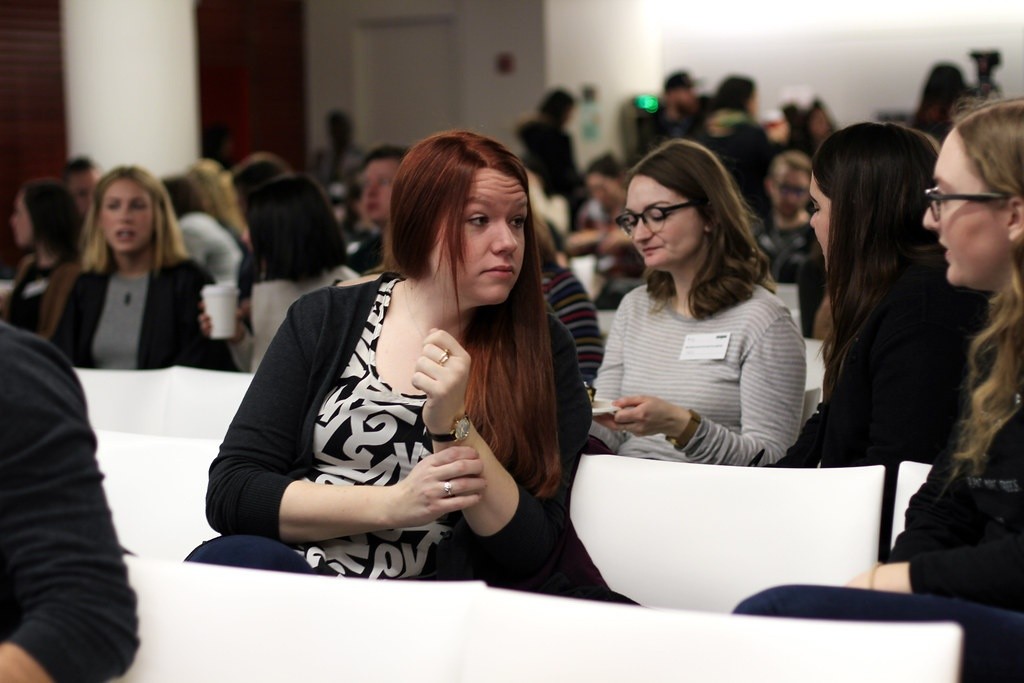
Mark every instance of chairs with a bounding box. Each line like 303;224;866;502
65;253;966;683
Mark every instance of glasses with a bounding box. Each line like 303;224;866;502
614;199;700;237
919;186;1013;222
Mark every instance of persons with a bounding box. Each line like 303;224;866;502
0;323;140;683
182;131;640;605
585;141;807;466
0;156;101;339
764;123;994;564
198;172;359;374
519;71;833;399
910;51;1001;142
732;98;1024;683
163;110;408;280
50;166;234;372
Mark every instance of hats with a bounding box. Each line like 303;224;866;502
664;72;697;90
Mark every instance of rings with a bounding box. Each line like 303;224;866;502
438;349;449;363
622;424;626;433
444;481;451;495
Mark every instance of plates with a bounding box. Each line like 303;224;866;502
592;399;619;415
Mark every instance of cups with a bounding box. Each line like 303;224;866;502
0;280;15;319
200;285;240;338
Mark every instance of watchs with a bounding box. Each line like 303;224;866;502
426;412;470;442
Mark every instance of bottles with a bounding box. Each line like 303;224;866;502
584;382;593;403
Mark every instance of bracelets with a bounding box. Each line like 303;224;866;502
666;409;701;447
